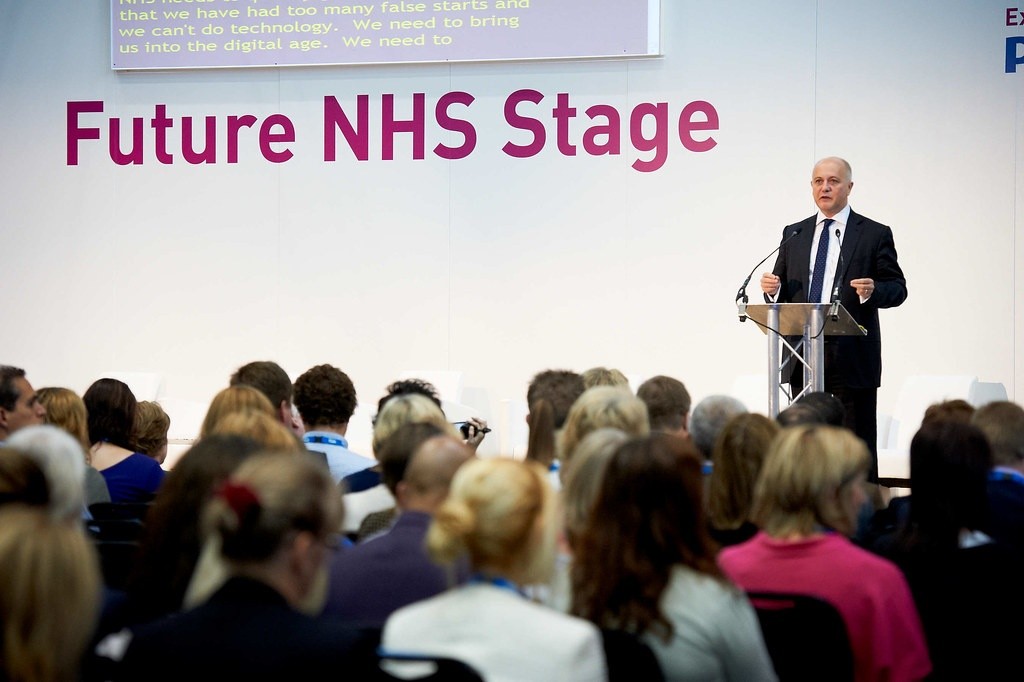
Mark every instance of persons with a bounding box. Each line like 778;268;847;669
761;156;908;481
0;361;1024;682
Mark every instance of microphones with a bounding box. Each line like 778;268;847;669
735;227;803;301
831;229;844;302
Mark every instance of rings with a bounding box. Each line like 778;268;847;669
865;290;867;294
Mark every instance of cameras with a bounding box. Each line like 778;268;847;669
453;422;478;440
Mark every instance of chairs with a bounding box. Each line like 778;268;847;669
86;502;857;682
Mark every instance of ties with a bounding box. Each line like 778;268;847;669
809;218;832;302
